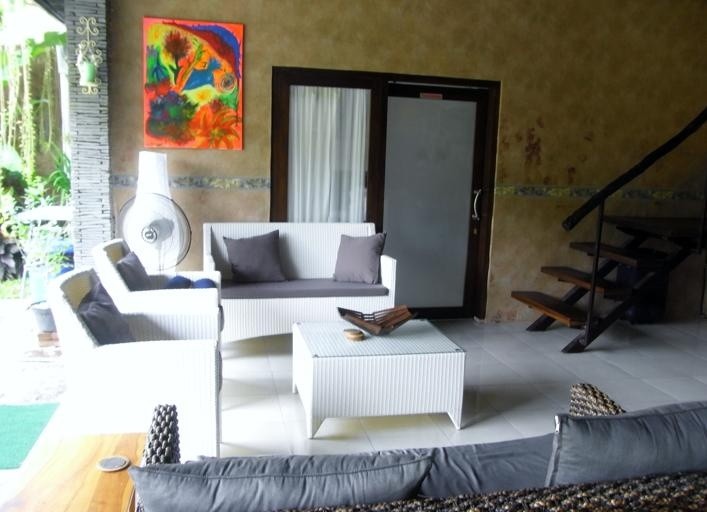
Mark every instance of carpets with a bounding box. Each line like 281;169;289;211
0;401;61;469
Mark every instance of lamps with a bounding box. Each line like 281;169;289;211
73;15;105;97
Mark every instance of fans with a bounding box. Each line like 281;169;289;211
114;192;192;274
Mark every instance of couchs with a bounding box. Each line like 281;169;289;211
129;381;706;512
203;221;398;345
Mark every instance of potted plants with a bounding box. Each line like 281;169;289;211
14;224;71;333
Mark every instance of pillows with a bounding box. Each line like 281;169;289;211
129;400;702;511
224;231;388;284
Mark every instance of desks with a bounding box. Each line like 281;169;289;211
0;398;146;512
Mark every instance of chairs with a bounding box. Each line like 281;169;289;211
43;237;224;391
0;204;73;302
